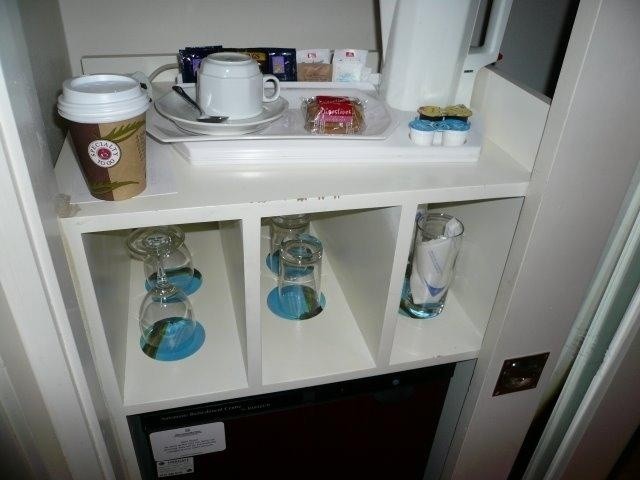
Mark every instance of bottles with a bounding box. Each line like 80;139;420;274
398;213;464;319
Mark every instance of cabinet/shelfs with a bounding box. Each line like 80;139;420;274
1;0;606;480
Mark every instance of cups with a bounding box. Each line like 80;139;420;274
379;1;513;110
405;106;472;147
56;73;151;201
269;214;322;316
197;50;281;119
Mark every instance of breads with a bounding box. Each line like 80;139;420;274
305;101;365;134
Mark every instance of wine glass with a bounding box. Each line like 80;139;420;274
123;224;197;348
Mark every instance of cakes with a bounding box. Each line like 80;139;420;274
409;103;472;131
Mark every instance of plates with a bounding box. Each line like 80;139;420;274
153;82;290;136
